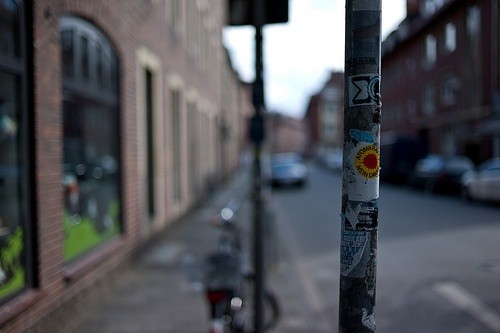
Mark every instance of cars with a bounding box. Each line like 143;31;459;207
321;151;500;204
269;152;306;188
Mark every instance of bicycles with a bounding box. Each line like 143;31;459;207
204;198;281;333
